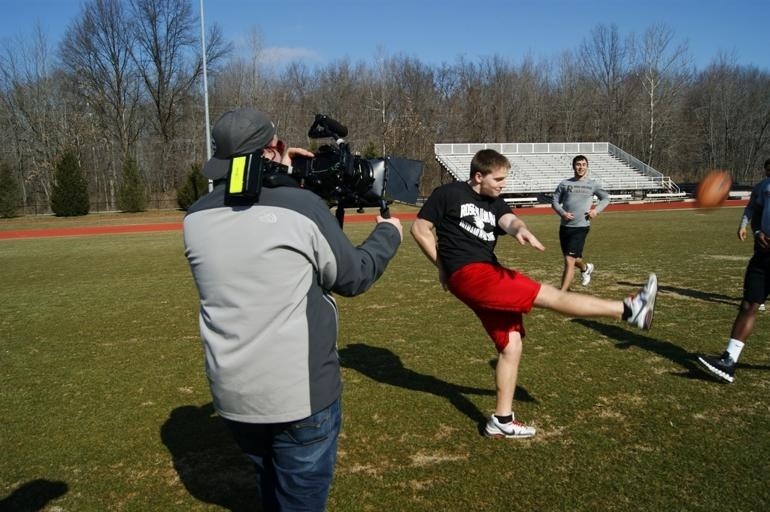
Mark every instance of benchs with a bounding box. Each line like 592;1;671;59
435;143;682;210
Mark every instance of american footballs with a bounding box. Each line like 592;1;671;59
698;168;732;211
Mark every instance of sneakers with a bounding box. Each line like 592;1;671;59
624;272;658;331
581;262;594;287
482;410;535;438
696;351;736;383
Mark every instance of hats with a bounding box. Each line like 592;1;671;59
198;108;276;180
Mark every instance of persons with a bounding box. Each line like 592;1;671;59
551;155;611;292
739;158;770;310
698;183;769;383
182;109;403;511
409;148;658;440
